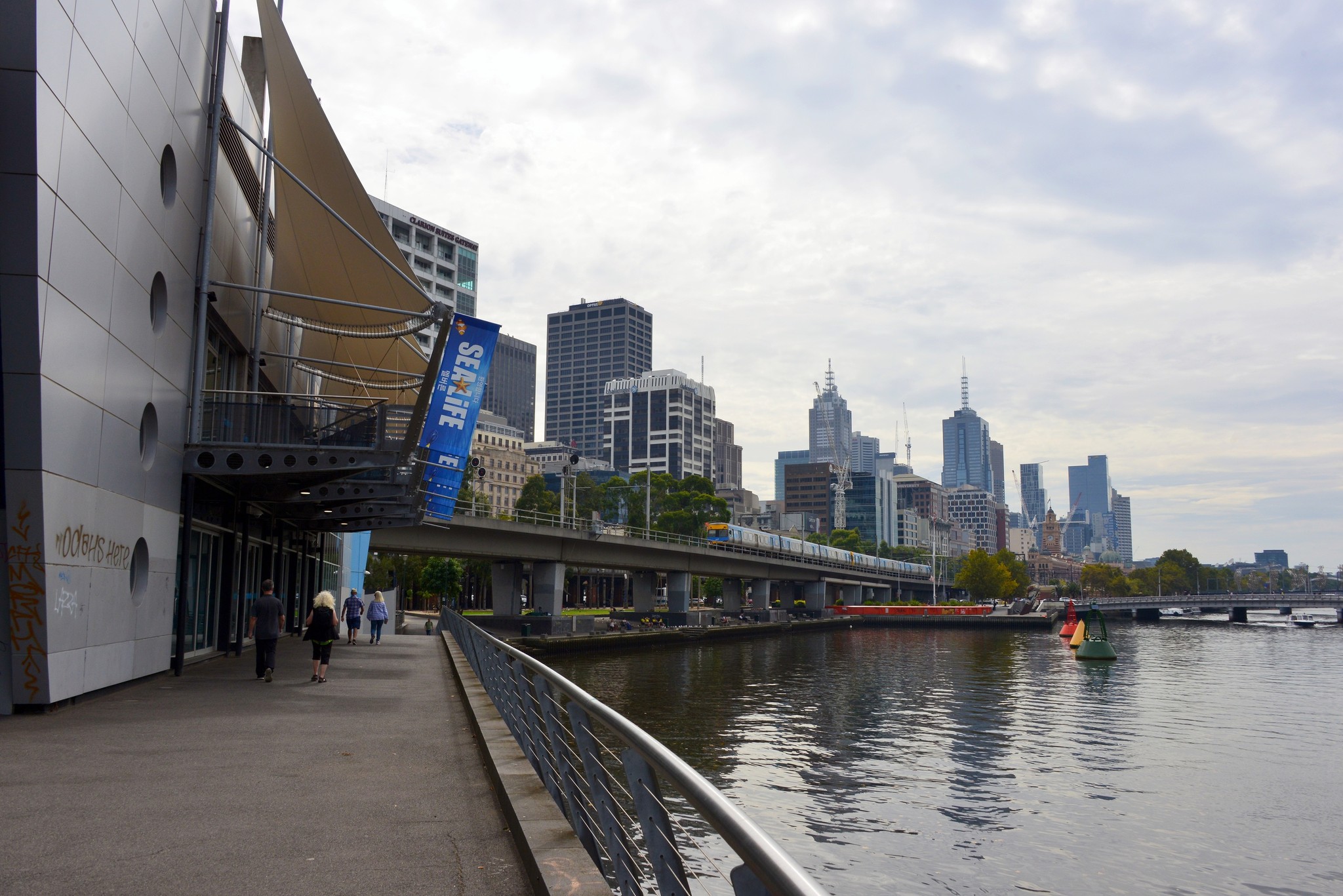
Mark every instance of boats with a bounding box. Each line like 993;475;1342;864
1284;613;1315;628
1159;608;1201;616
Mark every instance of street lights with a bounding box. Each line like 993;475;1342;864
890;561;900;601
601;569;605;606
926;559;960;602
443;557;450;605
401;554;408;612
1189;566;1199;595
1300;562;1310;594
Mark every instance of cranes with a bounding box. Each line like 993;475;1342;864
1011;469;1039;534
1060;491;1083;552
813;381;852;529
895;402;910;473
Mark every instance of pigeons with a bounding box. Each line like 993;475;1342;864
679;610;687;612
562;607;570;612
662;624;713;629
522;611;525;612
595;618;604;624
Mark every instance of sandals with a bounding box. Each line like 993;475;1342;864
311;675;318;680
318;676;327;682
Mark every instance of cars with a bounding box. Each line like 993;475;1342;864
977;598;1007;605
1040;597;1077;605
689;597;723;606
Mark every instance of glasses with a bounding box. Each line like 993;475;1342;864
374;594;376;596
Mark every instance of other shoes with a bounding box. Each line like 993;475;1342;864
265;668;272;682
257;676;264;679
348;641;351;644
370;635;375;644
376;641;381;644
352;640;357;645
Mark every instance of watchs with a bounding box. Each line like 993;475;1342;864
341;616;343;618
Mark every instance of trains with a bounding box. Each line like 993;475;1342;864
707;523;931;581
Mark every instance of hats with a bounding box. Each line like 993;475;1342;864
351;588;357;593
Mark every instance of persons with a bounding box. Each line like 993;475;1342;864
1313;592;1316;598
1251;591;1254;598
1230;591;1233;598
993;602;995;610
622;617;633;627
1318;592;1322;598
611;607;616;612
723;615;729;624
303;590;340;682
1272;590;1274;598
738;613;748;623
424;618;434;635
641;616;665;628
1281;590;1283;598
538;607;543;613
248;579;285;682
341;588;365;645
366;591;389;645
1014;598;1017;601
609;619;615;631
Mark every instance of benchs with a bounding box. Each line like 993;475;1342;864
716;617;735;624
606;622;619;631
641;622;660;628
803;613;810;618
740;616;754;622
788;614;796;619
619;621;633;630
